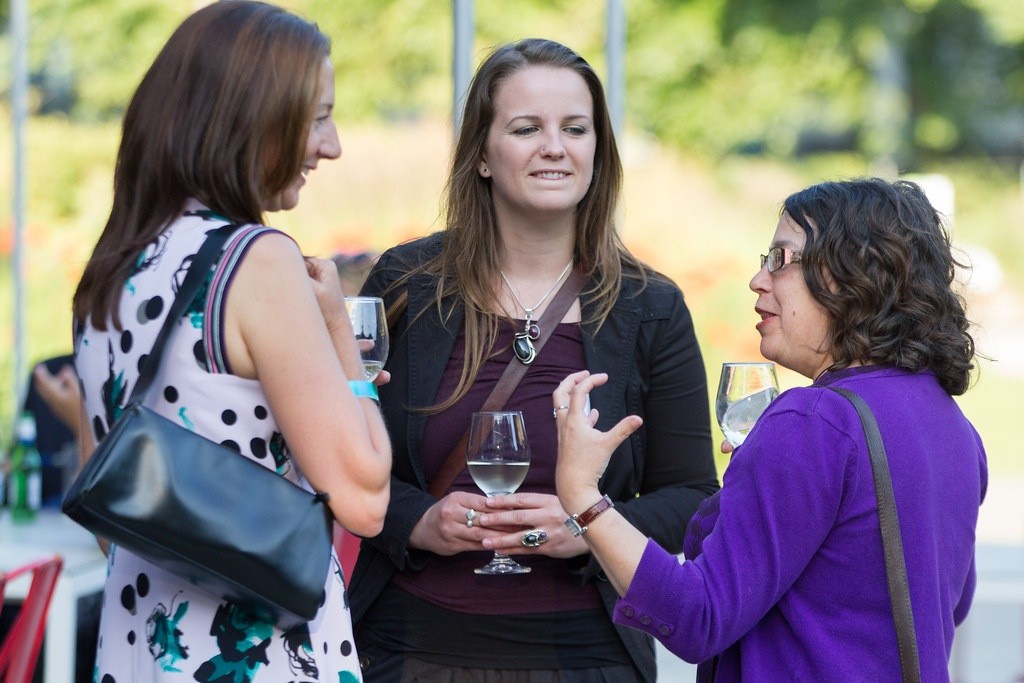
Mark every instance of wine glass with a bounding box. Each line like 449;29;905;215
467;411;532;574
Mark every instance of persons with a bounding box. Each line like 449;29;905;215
346;38;721;683
37;1;394;683
552;176;989;683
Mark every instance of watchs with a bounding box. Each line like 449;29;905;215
564;493;614;538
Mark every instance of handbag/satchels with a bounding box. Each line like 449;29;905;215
60;225;335;623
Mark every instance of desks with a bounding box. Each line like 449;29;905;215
0;511;108;683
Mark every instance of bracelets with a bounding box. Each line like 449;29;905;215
349;383;381;408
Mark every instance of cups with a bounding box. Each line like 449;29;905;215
343;296;389;383
715;362;780;448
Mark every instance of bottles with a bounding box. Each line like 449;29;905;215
7;409;42;525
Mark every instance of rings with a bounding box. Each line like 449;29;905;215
466;508;476;528
521;530;548;547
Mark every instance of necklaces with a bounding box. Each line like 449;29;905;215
501;258;574;365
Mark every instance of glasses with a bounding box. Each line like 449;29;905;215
760;247;826;273
